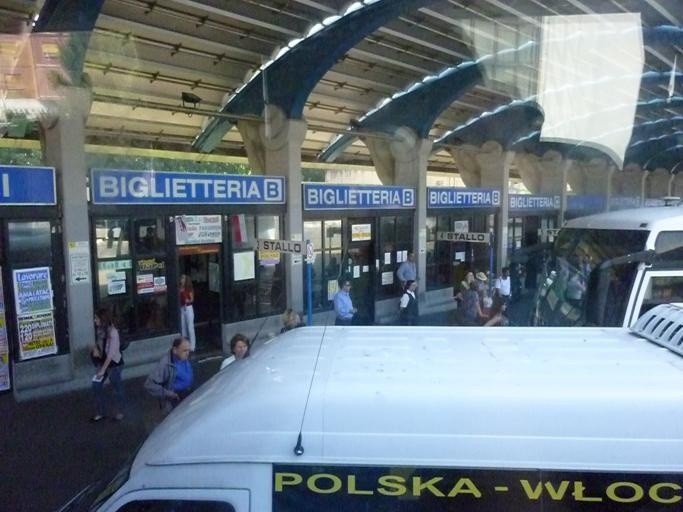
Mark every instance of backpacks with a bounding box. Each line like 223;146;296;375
116;323;130;351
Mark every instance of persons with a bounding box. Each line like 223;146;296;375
334;273;358;326
280;308;306;334
452;255;547;327
90;306;125;423
221;333;250;370
396;254;419;326
177;274;195;352
143;338;196;414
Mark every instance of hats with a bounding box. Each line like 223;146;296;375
475;272;488;281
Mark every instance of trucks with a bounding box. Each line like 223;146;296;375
57;223;683;509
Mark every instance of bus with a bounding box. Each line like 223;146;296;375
522;196;683;330
